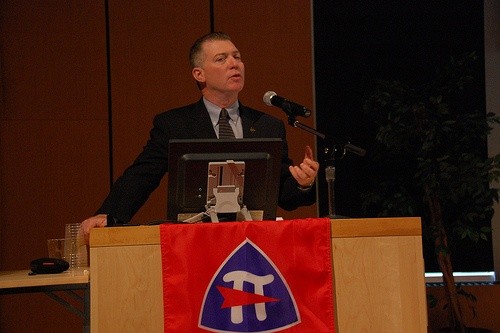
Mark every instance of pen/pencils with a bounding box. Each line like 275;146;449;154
123;224;139;226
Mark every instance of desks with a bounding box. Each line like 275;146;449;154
0;269;90;333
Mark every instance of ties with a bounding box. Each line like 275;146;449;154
218;107;236;140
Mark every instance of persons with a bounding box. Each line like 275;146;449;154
78;33;320;243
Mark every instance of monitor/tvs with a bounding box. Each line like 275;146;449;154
166;138;282;221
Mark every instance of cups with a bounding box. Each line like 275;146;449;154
47;238;65;260
62;222;88;274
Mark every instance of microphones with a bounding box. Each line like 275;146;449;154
262;91;311;118
